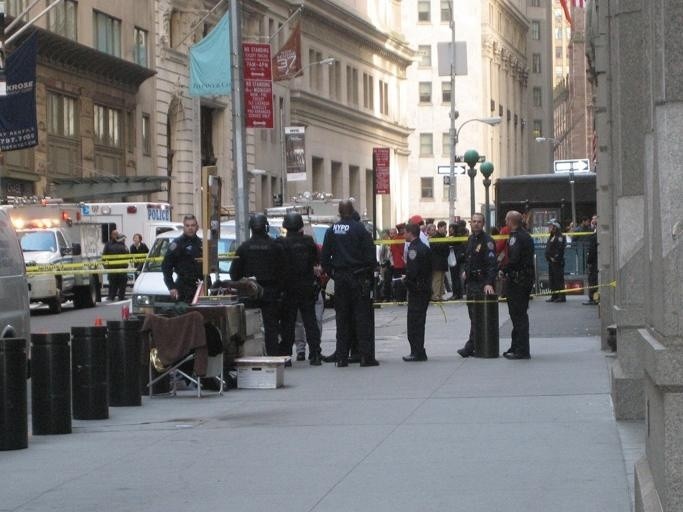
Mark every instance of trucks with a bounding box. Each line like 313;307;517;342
0;194;182;314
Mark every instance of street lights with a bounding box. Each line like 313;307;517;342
278;58;335;202
479;161;493;233
448;115;503;232
534;137;579;230
464;150;479;216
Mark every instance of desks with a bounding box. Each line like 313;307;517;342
155;303;244;390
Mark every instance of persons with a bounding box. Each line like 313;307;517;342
102;230;119;270
161;216;203;303
379;210;535;362
544;213;598;305
130;233;149;280
107;234;129;301
230;201;380;367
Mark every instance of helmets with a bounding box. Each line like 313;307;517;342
282;211;304;229
547;218;562;233
248;213;269;233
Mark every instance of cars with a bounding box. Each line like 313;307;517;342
130;211;382;319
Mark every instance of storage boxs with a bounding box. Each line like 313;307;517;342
239;308;262;342
234;356;291;388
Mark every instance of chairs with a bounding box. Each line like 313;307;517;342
145;312;226;399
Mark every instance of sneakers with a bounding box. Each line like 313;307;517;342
582;300;597;305
450;294;459;300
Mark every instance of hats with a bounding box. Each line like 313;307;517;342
409;215;423;224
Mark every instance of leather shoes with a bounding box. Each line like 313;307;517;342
361;356;379;365
458;346;472;357
339;356;348;366
298;353;305;360
503;351;530;359
546;296;551;302
325;353;338;360
403;350;427;362
554;296;565;301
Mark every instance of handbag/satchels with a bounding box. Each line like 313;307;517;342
355;267;376;294
